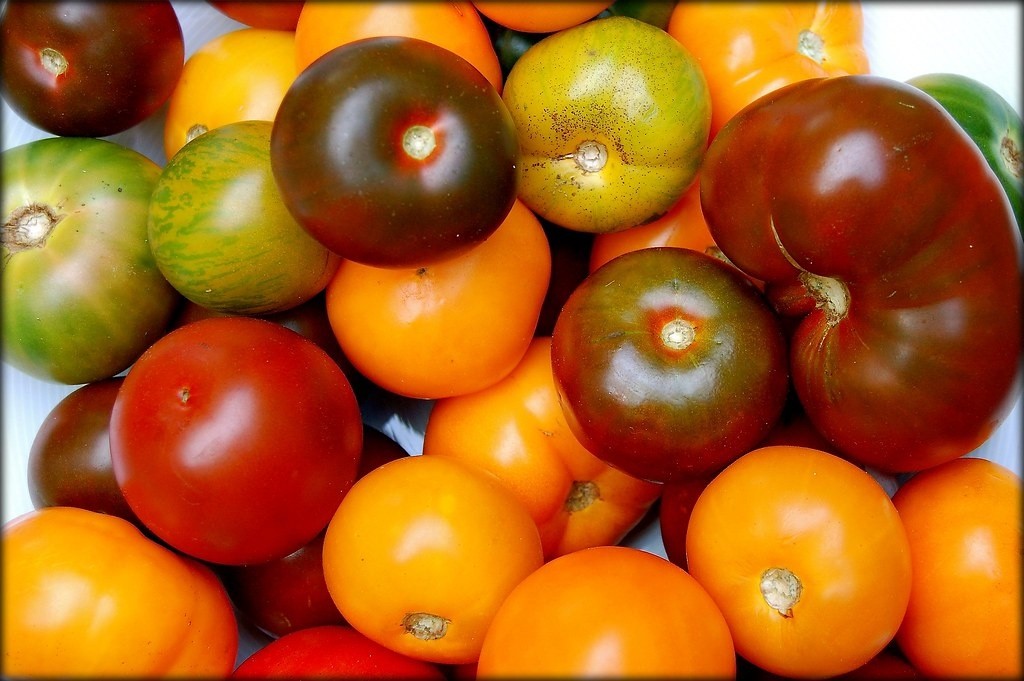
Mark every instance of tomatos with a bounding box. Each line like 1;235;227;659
0;0;1024;681
